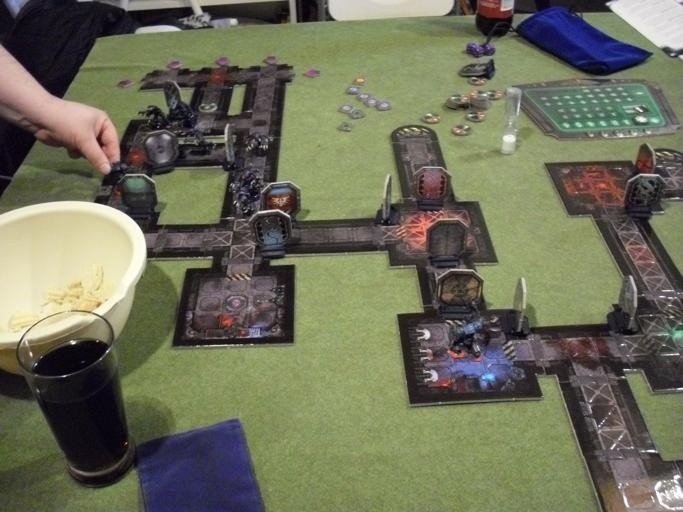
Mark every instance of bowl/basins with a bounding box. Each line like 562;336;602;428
0;199;150;380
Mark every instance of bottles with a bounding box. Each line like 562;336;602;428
475;1;514;38
500;86;521;154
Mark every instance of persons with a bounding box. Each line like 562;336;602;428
0;41;121;176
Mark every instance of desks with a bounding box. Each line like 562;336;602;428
0;10;683;512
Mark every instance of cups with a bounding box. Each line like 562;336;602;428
15;308;135;489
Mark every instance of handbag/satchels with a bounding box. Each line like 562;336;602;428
516;7;653;75
